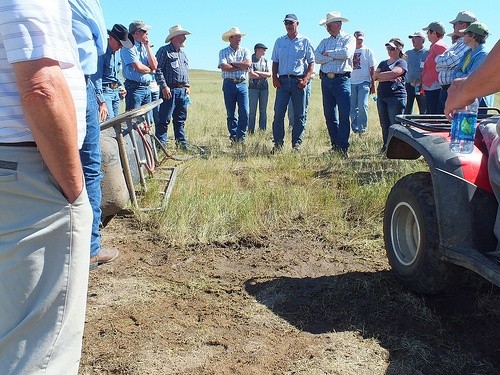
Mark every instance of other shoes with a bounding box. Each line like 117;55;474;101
89;247;119;272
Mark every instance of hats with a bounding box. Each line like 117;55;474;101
354;30;365;40
107;24;135;49
318;11;348;26
408;31;426;39
385;38;405;50
459;23;490;37
254;43;268;49
283;14;298;22
165;24;190;43
446;32;457;38
423;21;445;36
128;20;150;32
222;26;245;42
449;10;478;25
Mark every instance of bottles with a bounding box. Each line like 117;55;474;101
370;91;378;103
447;74;479;154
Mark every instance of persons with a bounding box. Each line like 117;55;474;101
248;44;271;136
154;25;191;151
451;22;495;117
371;38;409;158
350;31;375;137
1;0;94;375
443;39;500;123
270;13;315;154
65;0;120;271
434;10;477;114
314;11;357;159
90;24;135;122
119;21;158;130
287;56;315;135
422;22;449;114
403;31;429;120
219;27;252;147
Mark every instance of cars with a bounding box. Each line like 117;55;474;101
382;104;499;296
